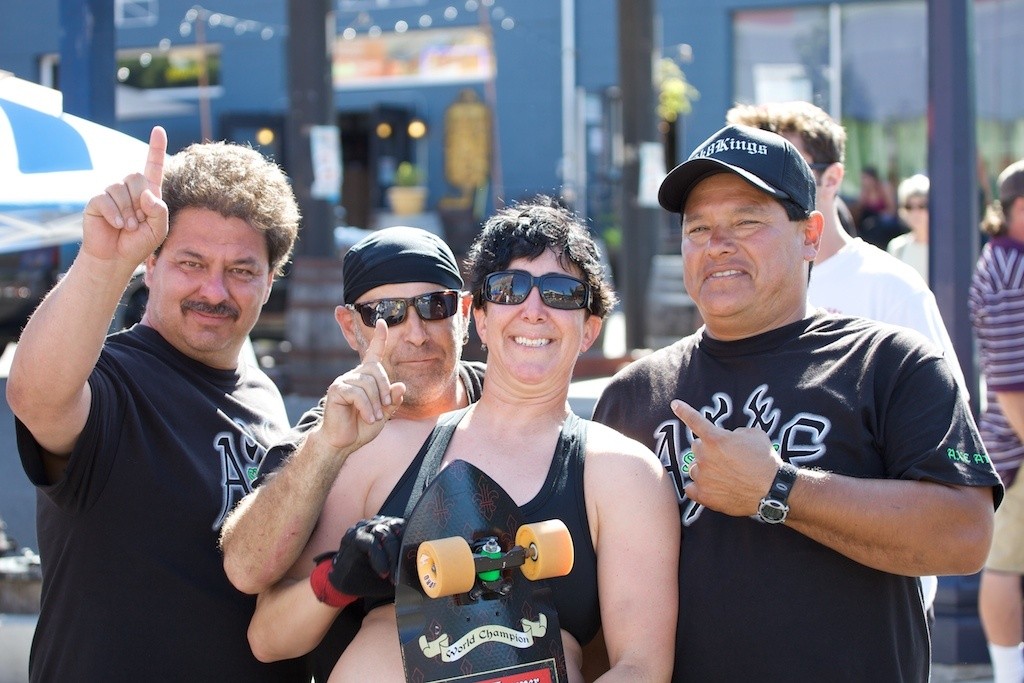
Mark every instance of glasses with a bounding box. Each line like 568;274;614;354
475;269;595;313
344;289;462;327
898;201;930;212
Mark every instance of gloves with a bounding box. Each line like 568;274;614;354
312;513;407;607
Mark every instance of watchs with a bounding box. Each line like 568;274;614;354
758;464;799;524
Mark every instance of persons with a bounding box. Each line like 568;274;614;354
730;100;969;400
246;207;682;683
593;124;1005;683
855;166;928;283
222;227;486;593
6;126;297;683
970;160;1024;683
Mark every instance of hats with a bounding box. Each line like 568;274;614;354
341;225;464;301
658;124;816;216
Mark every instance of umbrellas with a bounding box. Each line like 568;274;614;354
0;70;171;254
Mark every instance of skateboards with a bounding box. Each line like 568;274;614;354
393;459;575;683
